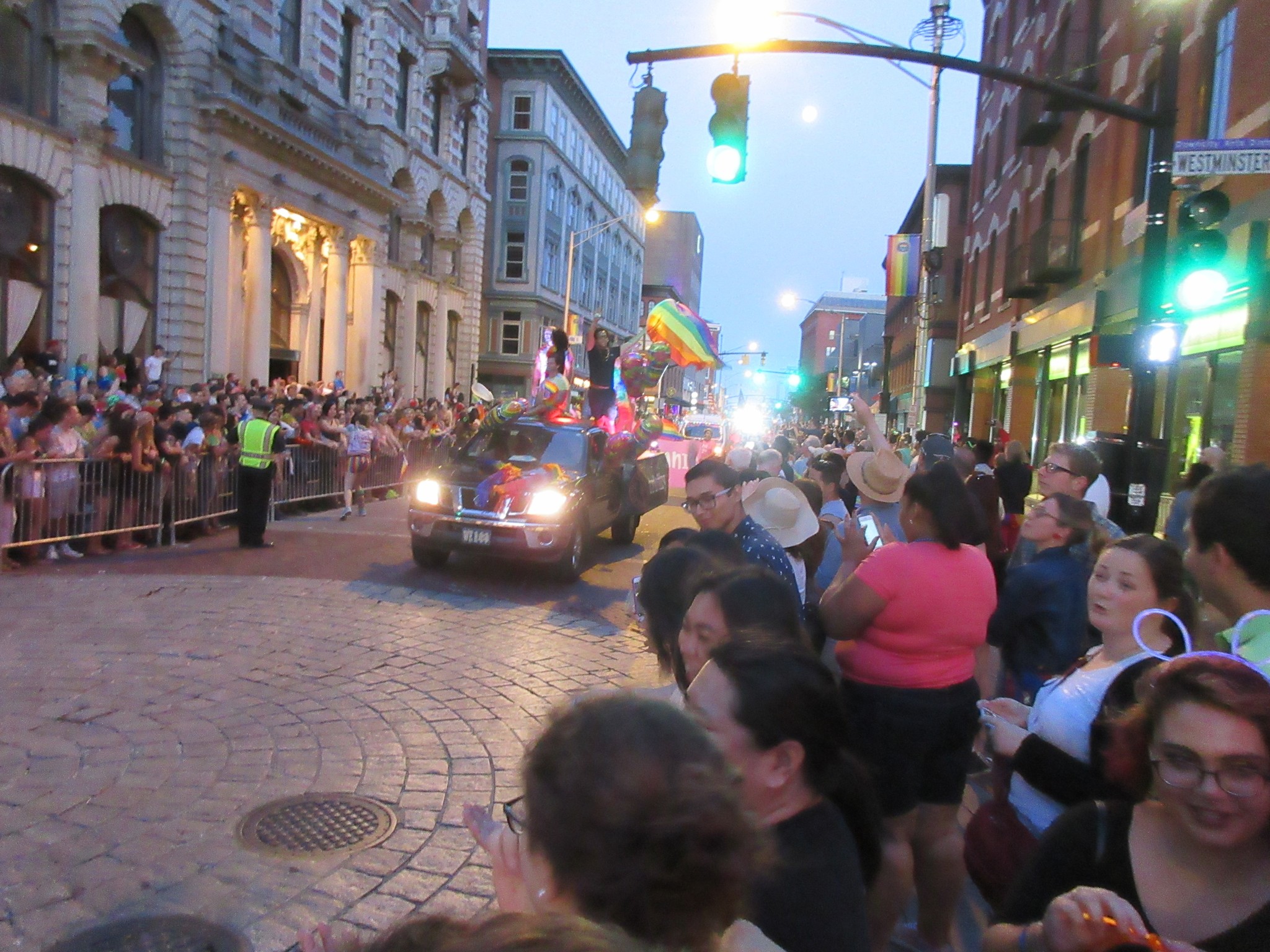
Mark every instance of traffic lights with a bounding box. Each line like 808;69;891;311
789;375;800;385
708;73;742;182
775;403;782;408
1138;326;1179;363
1180;189;1229;312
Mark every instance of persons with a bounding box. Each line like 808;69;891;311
2;324;1231;710
978;529;1199;924
1181;459;1267;683
978;650;1269;952
678;625;876;952
464;680;797;952
820;467;998;951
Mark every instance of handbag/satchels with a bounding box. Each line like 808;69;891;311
962;800;1045;915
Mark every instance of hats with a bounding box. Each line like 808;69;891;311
846;450;913;503
741;476;820;549
808;446;828;458
919;435;955;473
724;447;752;472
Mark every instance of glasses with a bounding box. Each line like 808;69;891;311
503;794;533;835
1029;503;1058;521
681;487;732;513
1040;461;1080;477
1147;746;1269;797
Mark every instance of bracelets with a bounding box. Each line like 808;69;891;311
1016;922;1037;952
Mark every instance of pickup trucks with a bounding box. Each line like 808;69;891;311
677;415;733;464
409;414;670;582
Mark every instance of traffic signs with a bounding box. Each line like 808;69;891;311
1170;138;1270;176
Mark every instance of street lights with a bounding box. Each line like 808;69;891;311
710;2;964;449
724;370;752;398
781;293;845;434
563;209;661;333
717;359;743;410
658;351;768;418
755;370;851;429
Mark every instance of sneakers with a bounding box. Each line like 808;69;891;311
889;923;955;952
46;541;148;561
359;510;367;516
340;506;352;521
